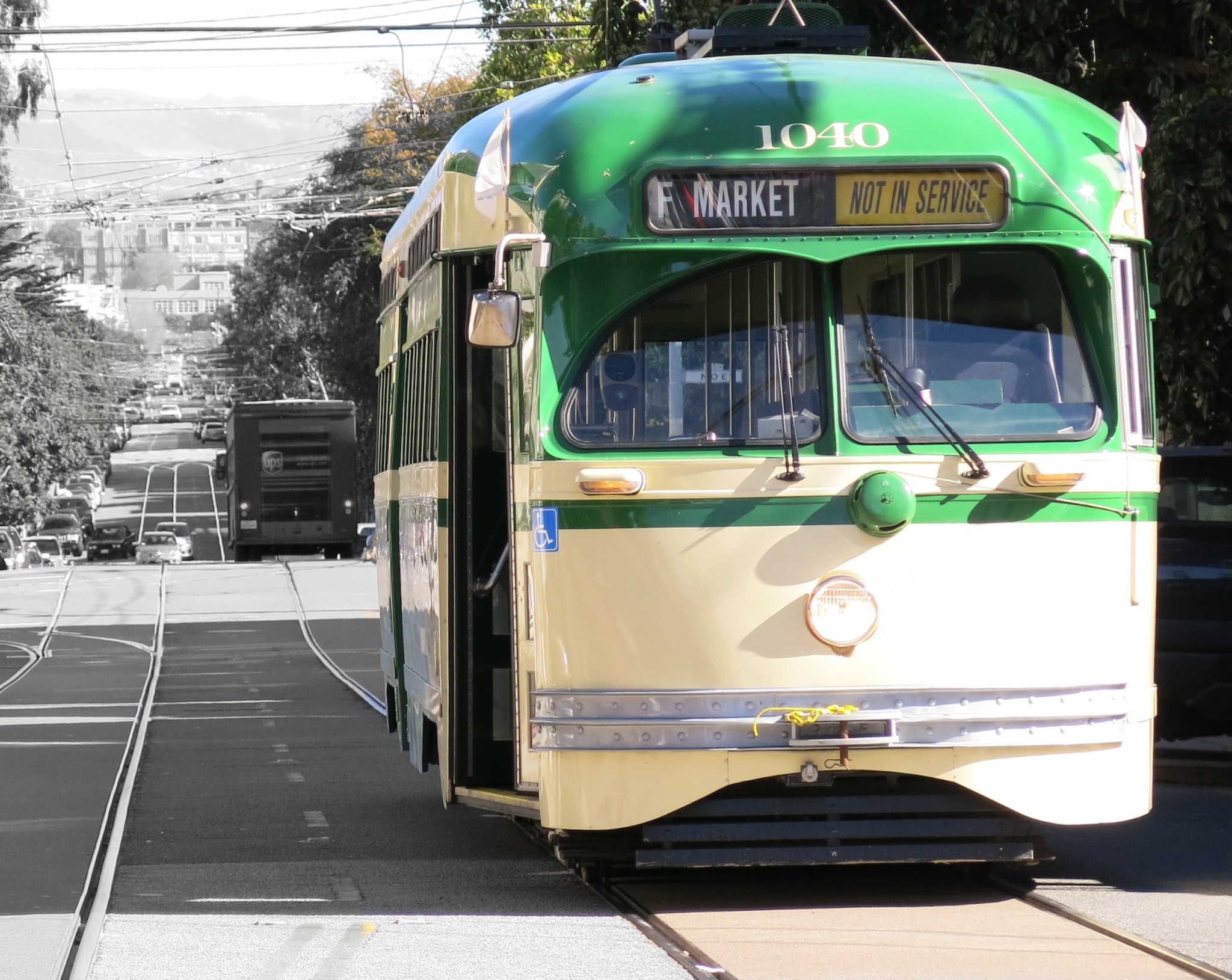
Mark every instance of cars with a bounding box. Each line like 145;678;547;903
0;449;111;570
155;522;197;560
192;415;227;444
157;403;182;423
132;531;182;565
107;374;185;451
87;524;136;563
181;356;206;400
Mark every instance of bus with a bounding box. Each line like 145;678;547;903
372;4;1164;877
215;397;360;563
